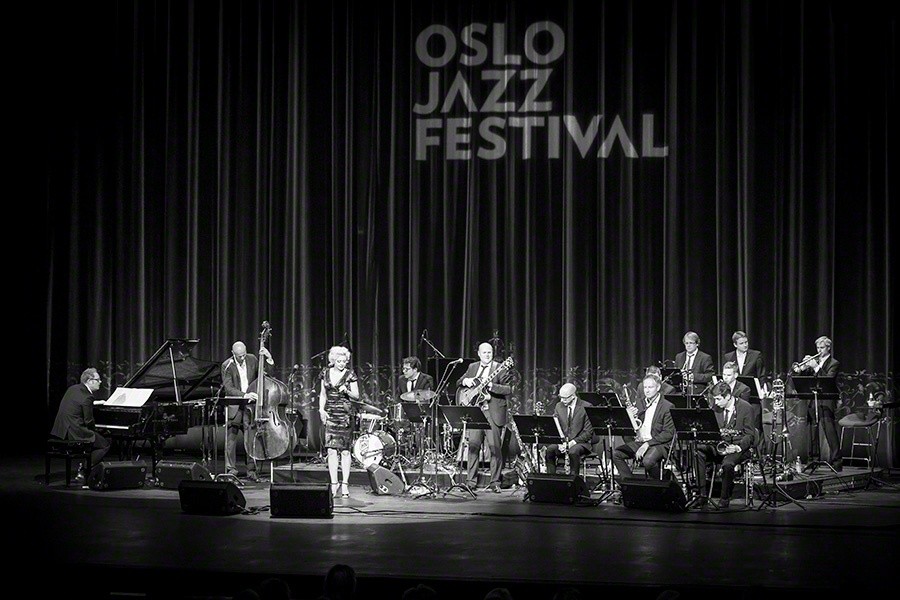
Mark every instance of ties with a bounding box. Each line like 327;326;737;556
724;409;730;426
568;407;572;428
411;379;416;391
477;365;489;378
687;354;694;371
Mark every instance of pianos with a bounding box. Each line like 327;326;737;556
93;337;226;442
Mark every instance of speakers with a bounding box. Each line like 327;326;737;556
154;458;215;489
178;478;247;516
524;472;591;505
88;459;147;491
620;477;687;513
366;463;404;495
269;481;334;520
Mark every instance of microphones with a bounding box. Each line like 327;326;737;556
449;357;464;364
288;363;299;382
344;332;353;354
417;330;426;350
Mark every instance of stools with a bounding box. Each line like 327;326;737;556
46;436;91;487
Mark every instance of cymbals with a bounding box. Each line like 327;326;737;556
399;387;436;402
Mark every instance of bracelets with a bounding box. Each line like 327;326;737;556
346;389;347;394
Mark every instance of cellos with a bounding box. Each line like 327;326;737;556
241;320;299;462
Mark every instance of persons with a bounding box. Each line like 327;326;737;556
722;330;766;388
675;331;714;395
455;342;511;493
694;382;756;509
220;341;275;479
319;344;360;499
544;383;593;476
396;357;433;459
635;364;676;400
721;360;752;402
49;367;112;479
611;374;675;480
793;336;843;473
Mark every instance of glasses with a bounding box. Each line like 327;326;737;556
684;341;696;345
402;367;412;371
90;378;100;382
558;394;574;399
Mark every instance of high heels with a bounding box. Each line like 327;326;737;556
341;481;350;498
329;481;341;498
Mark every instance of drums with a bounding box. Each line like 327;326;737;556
352;430;397;468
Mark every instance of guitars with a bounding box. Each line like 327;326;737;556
455;355;515;406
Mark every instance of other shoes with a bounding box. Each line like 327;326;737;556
492;485;502;493
831;464;843;472
249;473;261;482
718;498;730;508
460;485;476;492
690;496;709;508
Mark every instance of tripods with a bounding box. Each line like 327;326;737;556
590;426;624;507
757;436;810;512
681;432;719;511
837;418;899;492
213;405;246;487
801;393;837;475
389;408;479;500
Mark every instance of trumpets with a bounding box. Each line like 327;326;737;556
791;351;822;374
